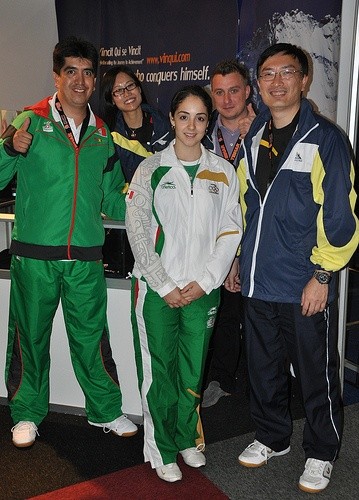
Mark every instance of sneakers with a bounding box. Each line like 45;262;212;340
298;457;334;494
88;413;138;437
11;420;40;447
155;462;182;483
179;443;206;468
237;438;290;468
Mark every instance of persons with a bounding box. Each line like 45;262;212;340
123;85;243;482
224;42;359;494
99;66;175;185
0;35;139;447
202;62;264;398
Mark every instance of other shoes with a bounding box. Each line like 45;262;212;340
202;381;231;407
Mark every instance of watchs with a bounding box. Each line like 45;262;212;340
313;270;332;284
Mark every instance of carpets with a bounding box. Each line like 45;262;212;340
28;463;231;500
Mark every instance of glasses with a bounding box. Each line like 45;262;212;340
112;82;138;97
259;67;302;81
